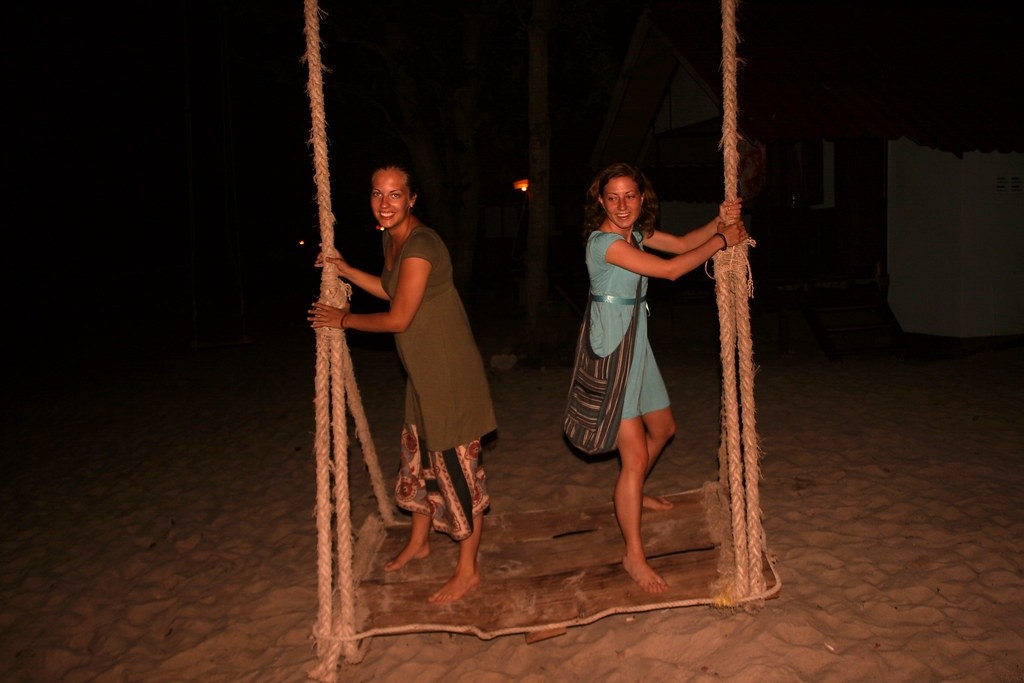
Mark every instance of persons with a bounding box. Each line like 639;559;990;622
581;165;748;593
307;162;497;603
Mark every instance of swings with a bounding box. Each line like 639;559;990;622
184;36;255;349
310;0;780;683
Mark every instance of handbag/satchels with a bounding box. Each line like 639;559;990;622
564;314;639;454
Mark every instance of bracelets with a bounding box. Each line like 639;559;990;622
714;233;727;250
340;313;348;328
